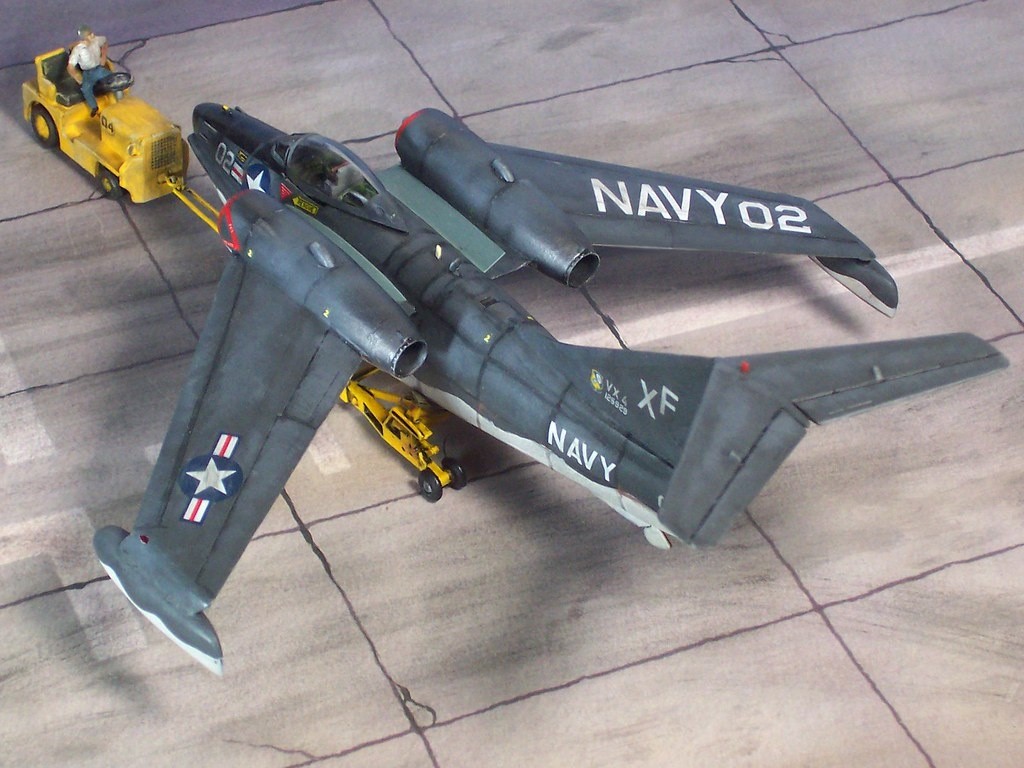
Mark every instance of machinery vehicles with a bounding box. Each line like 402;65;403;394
20;41;191;204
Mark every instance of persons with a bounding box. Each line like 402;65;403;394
67;23;124;118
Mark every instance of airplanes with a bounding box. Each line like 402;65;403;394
92;102;1010;681
